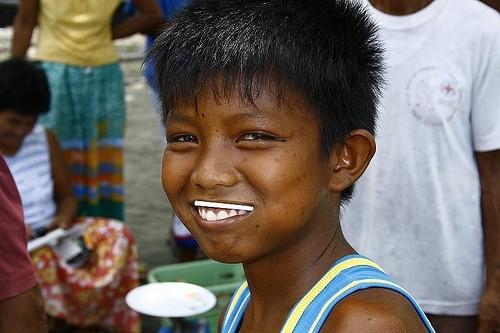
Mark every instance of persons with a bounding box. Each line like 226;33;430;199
0;0;187;333
339;0;500;333
142;0;438;333
